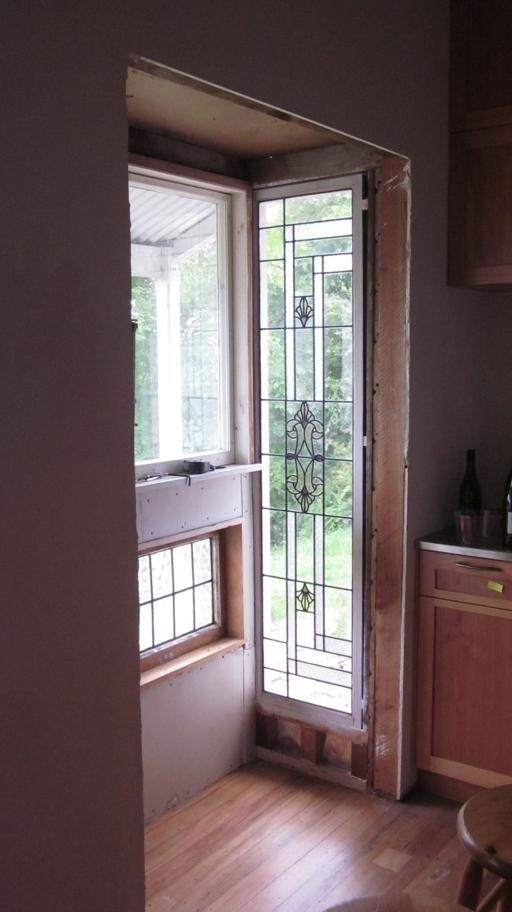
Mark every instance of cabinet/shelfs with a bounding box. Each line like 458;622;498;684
412;539;512;803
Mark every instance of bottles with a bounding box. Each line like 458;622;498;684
502;470;512;550
458;448;484;543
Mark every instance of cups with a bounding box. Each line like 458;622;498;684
454;510;491;544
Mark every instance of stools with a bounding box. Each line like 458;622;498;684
456;784;511;912
